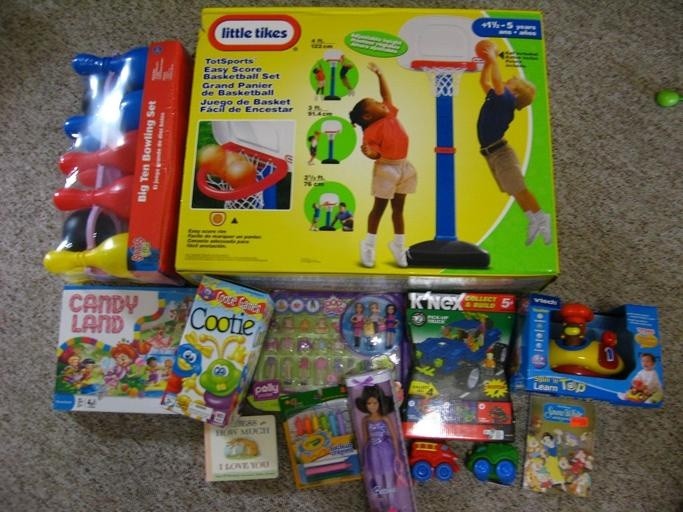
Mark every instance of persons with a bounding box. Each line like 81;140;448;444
349;64;418;267
618;350;663;405
313;63;327;101
330;203;354;230
307;133;317;166
309;204;321;231
338;58;355;98
477;45;551;247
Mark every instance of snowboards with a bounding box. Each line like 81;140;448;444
475;40;496;62
226;159;258;189
219;150;245;181
198;144;225;174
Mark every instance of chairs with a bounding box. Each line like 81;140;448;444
388;234;409;267
360;232;377;267
524;211;539;246
533;210;552;246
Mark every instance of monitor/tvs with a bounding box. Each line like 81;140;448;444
522;393;595;496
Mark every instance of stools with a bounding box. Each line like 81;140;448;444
480;139;508;155
